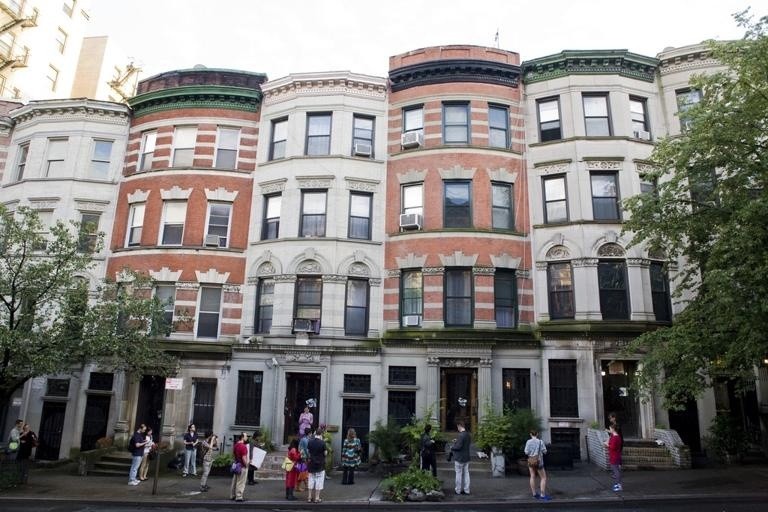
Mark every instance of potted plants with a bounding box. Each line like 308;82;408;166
508;408;544;476
212;455;233;477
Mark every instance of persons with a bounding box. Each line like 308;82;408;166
602;413;623;491
6;420;27;461
451;421;471;494
128;424;364;503
524;430;553;500
17;424;37;484
299;405;314;437
422;425;437;489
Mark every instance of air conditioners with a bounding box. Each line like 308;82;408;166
401;131;423;148
205;234;220;247
399;213;420;226
637;130;650;141
355;143;372;156
405;315;420;328
293;317;318;333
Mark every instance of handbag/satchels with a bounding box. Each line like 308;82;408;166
197;445;205;459
527;455;539;467
230;462;242;475
5;440;19;454
31;437;38;447
148;448;157;460
128;437;136;453
295;461;307;473
422;445;429;458
281;457;294;472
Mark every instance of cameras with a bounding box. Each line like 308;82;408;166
447;449;454;460
212;434;218;439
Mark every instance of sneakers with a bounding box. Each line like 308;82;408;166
182;473;198;478
200;485;210;492
128;476;148;485
610;470;623;491
532;493;552;501
285;488;321;502
230;496;247;502
341;480;354;485
248;480;258;485
455;490;470;495
